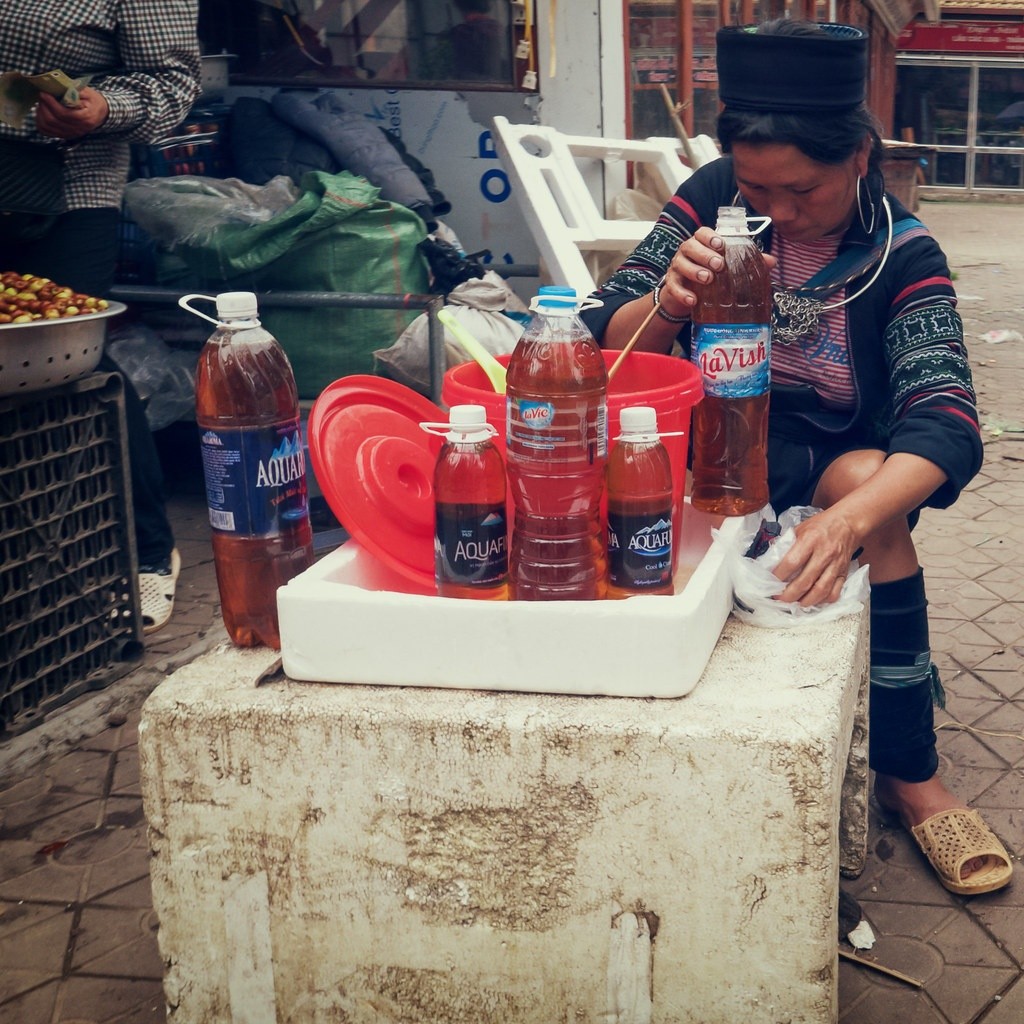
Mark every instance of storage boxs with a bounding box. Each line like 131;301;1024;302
273;495;758;701
0;371;145;737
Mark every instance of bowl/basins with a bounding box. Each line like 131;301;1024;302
0;298;129;396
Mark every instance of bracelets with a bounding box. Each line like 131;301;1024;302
653;273;685;322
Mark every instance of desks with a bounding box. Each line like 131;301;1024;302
132;554;873;1023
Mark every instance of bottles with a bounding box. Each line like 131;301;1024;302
503;284;609;601
605;405;686;601
417;403;507;600
174;289;314;651
691;200;778;518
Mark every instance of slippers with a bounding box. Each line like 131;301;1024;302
138;547;181;635
900;796;1013;895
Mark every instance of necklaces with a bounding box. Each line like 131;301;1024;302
731;189;893;347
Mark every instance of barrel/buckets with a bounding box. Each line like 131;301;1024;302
440;349;703;582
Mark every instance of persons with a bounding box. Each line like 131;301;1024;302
579;17;1013;895
1;0;204;634
439;0;502;82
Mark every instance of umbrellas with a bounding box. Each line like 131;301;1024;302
997;102;1024;118
